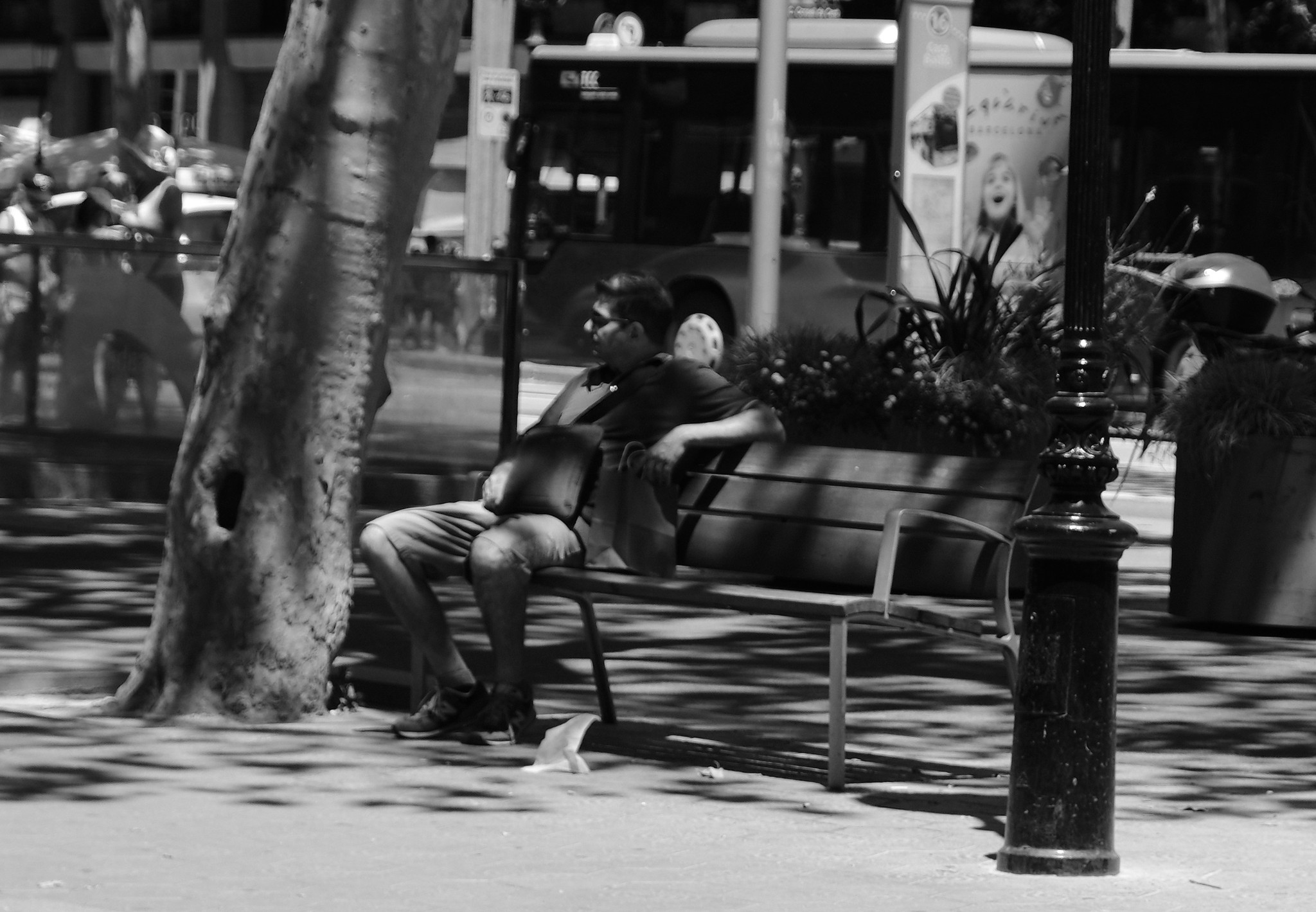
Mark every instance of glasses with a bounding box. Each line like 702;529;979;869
590;309;631;328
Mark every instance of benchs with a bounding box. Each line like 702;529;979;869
403;438;1046;789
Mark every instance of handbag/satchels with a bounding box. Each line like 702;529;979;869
480;424;604;526
582;441;679;579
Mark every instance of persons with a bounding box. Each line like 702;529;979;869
964;150;1051;294
0;99;195;431
360;271;787;749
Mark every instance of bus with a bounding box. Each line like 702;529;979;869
502;17;1075;364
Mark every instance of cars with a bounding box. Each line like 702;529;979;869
411;136;471;253
0;124;246;377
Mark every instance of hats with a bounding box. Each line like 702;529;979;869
117;123;180;175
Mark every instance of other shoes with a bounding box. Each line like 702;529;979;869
467;680;536;745
390;679;490;738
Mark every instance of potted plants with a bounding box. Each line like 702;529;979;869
1169;348;1316;624
